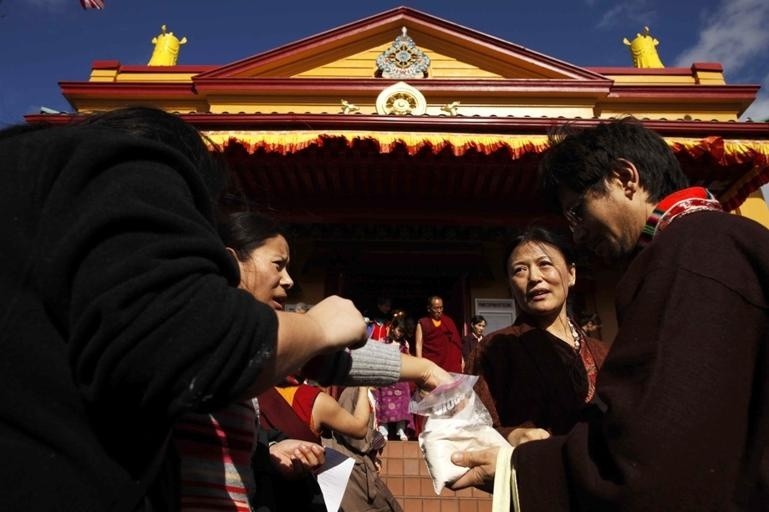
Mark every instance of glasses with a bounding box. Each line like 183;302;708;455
565;184;594;233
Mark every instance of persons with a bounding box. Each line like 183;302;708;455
0;105;369;512
463;225;611;448
248;293;489;511
445;121;769;511
575;311;604;337
176;207;468;511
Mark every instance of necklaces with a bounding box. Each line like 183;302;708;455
567;318;582;354
